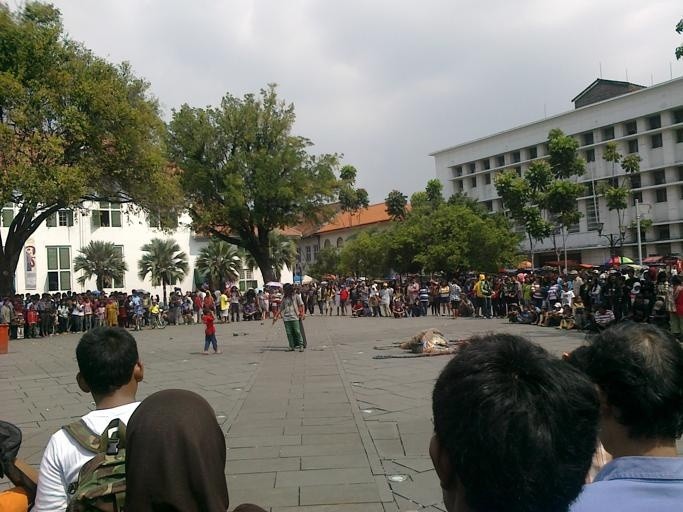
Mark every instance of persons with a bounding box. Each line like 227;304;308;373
561;344;617;483
118;387;267;512
568;321;681;511
200;307;222;355
271;283;306;352
30;324;144;512
427;330;607;512
0;418;38;512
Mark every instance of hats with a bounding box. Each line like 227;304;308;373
591;269;616;278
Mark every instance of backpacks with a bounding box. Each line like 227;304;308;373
61;419;126;511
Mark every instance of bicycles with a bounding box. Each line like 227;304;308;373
131;311;169;329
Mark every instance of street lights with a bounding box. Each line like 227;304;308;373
634;199;655;266
596;220;628;262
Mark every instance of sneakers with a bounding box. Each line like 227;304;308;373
201;351;209;355
214;350;221;354
285;347;294;352
299;346;304;352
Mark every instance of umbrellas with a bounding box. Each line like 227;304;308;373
655;254;681;271
516;260;532;270
608;256;633;267
641;256;662;265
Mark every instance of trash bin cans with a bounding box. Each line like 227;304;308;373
0;324;10;354
